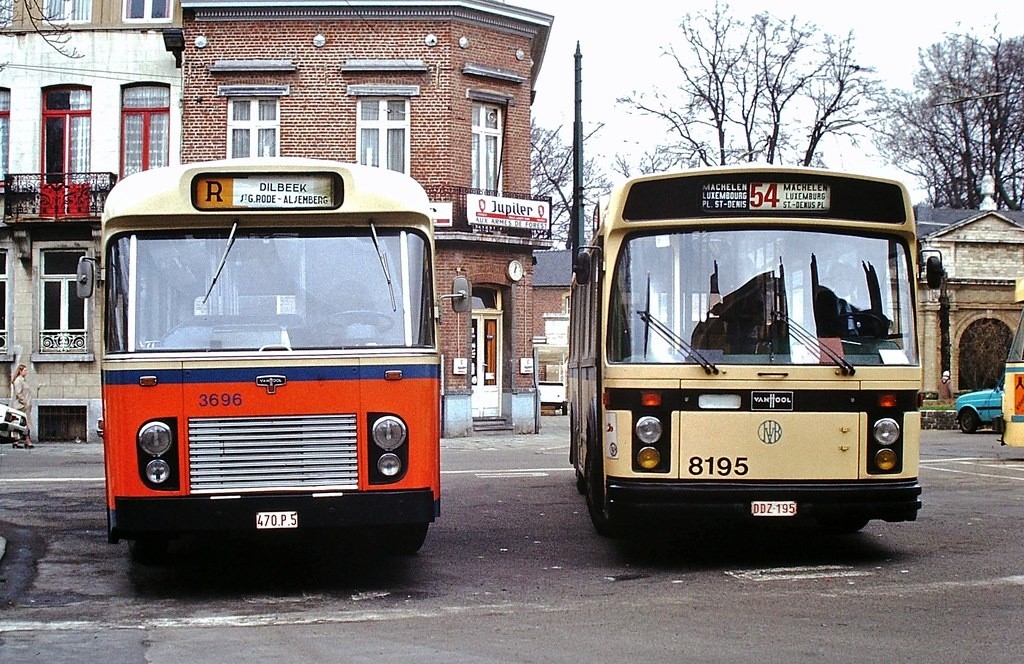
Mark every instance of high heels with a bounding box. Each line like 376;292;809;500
25;440;34;449
13;442;23;449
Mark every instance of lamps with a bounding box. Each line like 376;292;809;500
162;28;186;68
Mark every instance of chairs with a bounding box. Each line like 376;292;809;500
325;281;381;328
655;318;788;355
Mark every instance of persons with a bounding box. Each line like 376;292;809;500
813;264;880;338
11;364;34;449
937;371;954;400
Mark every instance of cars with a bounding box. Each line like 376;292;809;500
955;370;1008;433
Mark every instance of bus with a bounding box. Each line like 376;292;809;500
567;164;924;540
76;157;472;566
997;276;1024;449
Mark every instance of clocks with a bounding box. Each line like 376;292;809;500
505;260;524;283
